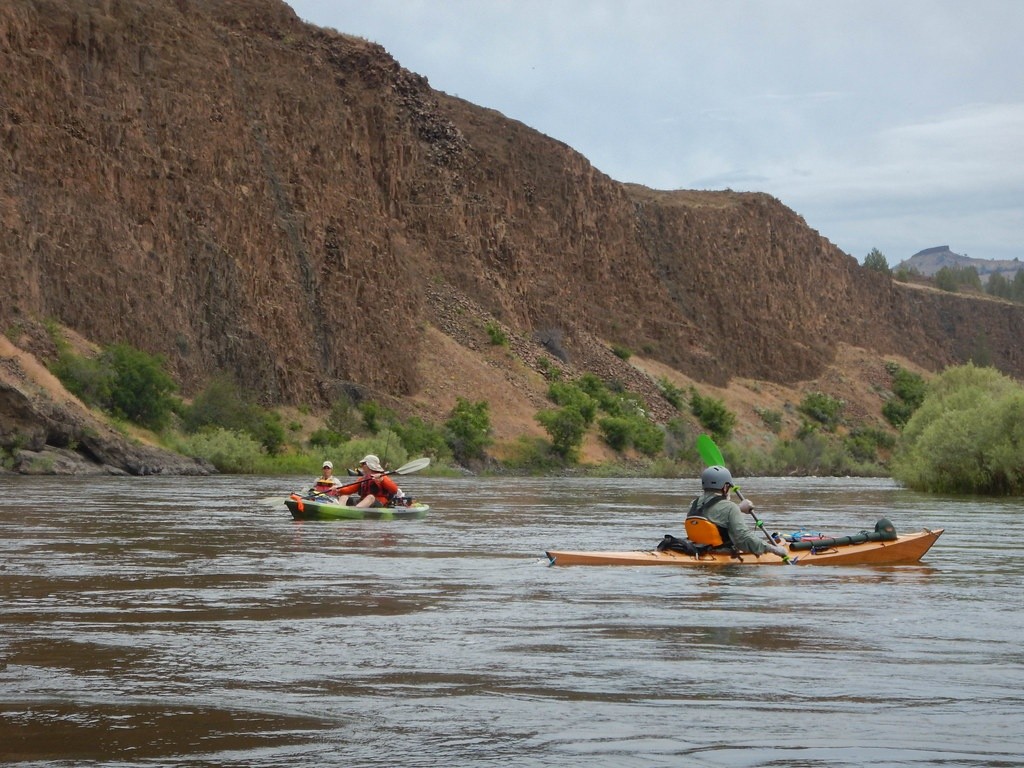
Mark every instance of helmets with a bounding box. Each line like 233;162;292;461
701;465;734;490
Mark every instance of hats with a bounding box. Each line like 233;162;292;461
359;454;385;472
323;461;333;469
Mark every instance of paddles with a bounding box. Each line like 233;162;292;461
696;433;793;564
300;457;431;498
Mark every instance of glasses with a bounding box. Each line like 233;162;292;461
323;467;331;470
362;463;366;466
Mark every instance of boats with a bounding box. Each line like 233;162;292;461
283;494;430;519
545;518;944;565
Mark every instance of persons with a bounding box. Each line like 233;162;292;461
329;455;404;509
307;460;343;506
686;466;789;557
